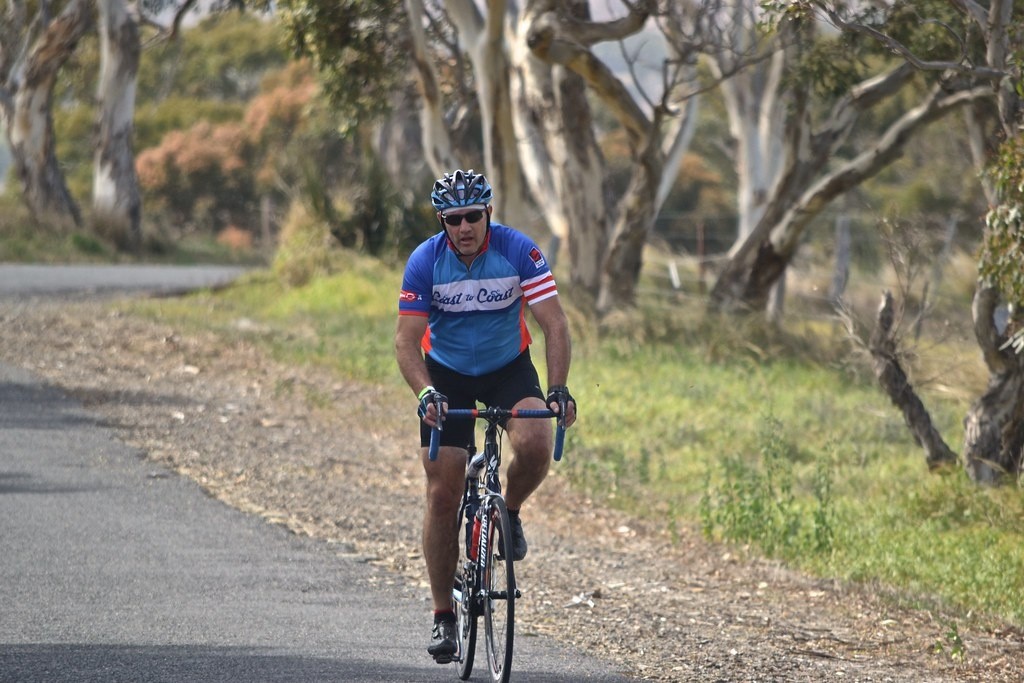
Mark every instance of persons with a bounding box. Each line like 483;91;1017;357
393;170;577;655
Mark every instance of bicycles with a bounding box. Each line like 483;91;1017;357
429;391;567;683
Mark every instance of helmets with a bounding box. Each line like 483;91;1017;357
432;169;494;211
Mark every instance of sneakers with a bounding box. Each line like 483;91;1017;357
428;611;458;655
495;508;527;560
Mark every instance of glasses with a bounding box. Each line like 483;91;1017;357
441;209;485;225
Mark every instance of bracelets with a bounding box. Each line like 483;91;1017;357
546;385;569;397
418;386;435;400
